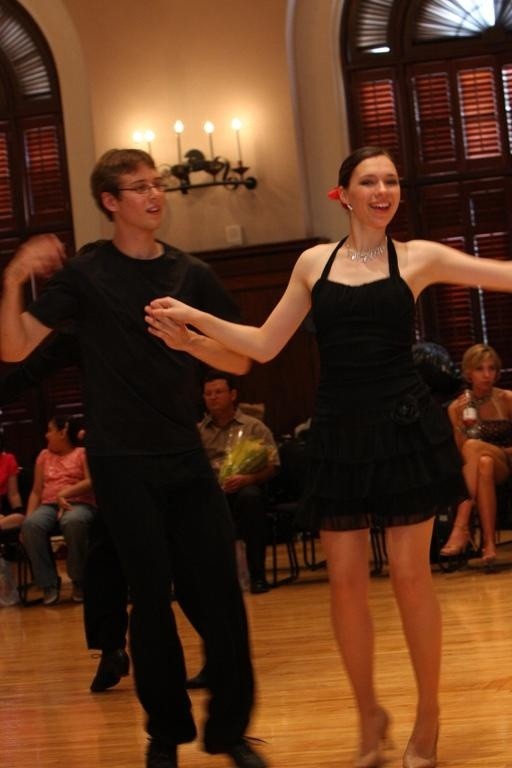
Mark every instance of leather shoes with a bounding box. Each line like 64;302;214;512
90;649;130;692
145;737;178;768
187;664;210;689
249;575;269;594
203;731;266;768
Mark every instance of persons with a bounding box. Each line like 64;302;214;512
442;346;511;569
196;370;281;595
149;148;512;768
20;413;96;604
0;148;268;768
0;427;25;602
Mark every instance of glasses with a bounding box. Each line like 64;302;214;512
203;389;232;398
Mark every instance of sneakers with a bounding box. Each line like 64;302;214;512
72;587;86;603
43;577;61;605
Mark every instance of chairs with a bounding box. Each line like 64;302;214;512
307;522;387;571
441;504;510;572
232;429;297;587
17;531;72;603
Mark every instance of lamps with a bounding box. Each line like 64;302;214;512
133;116;258;196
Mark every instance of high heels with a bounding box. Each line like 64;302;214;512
116;180;168;195
354;708;390;767
440;525;473;556
482;548;498;574
403;720;439;768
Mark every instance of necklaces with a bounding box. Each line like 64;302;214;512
343;236;387;263
470;389;493;407
